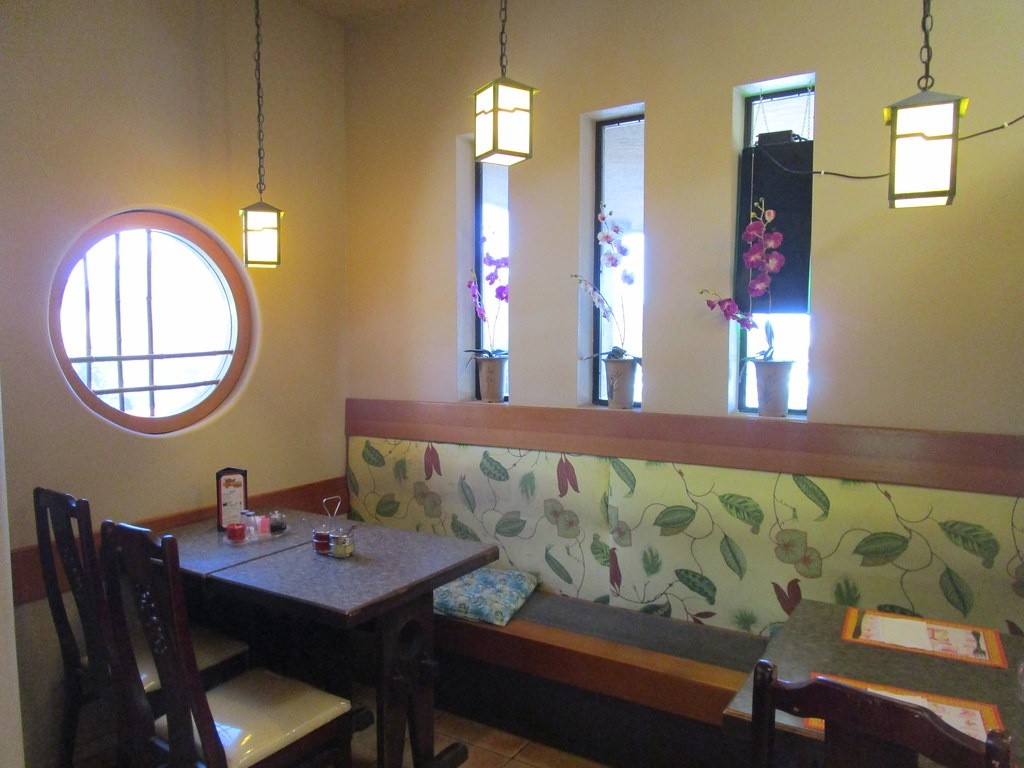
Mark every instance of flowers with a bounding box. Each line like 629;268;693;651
463;236;509;368
570;201;642;367
698;197;786;385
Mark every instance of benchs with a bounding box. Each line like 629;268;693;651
345;398;1024;764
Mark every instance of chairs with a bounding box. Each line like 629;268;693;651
98;517;375;768
31;486;252;768
749;660;1014;768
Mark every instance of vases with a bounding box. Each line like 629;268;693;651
752;359;794;418
473;355;507;401
602;359;637;408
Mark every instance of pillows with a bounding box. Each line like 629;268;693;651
432;562;538;627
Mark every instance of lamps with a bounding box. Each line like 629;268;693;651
886;3;970;211
473;0;538;168
238;0;284;270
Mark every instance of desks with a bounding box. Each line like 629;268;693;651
208;521;500;768
721;597;1023;767
115;502;364;626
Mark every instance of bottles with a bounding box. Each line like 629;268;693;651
225;509;287;545
313;525;353;559
